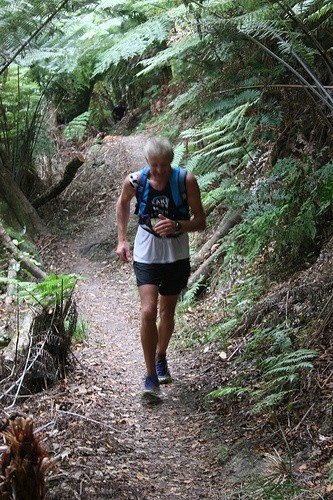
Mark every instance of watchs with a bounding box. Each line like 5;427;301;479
173;220;182;234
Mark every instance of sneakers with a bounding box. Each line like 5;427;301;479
142;376;160;401
155;358;172;383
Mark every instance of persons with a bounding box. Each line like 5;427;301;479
116;136;206;397
